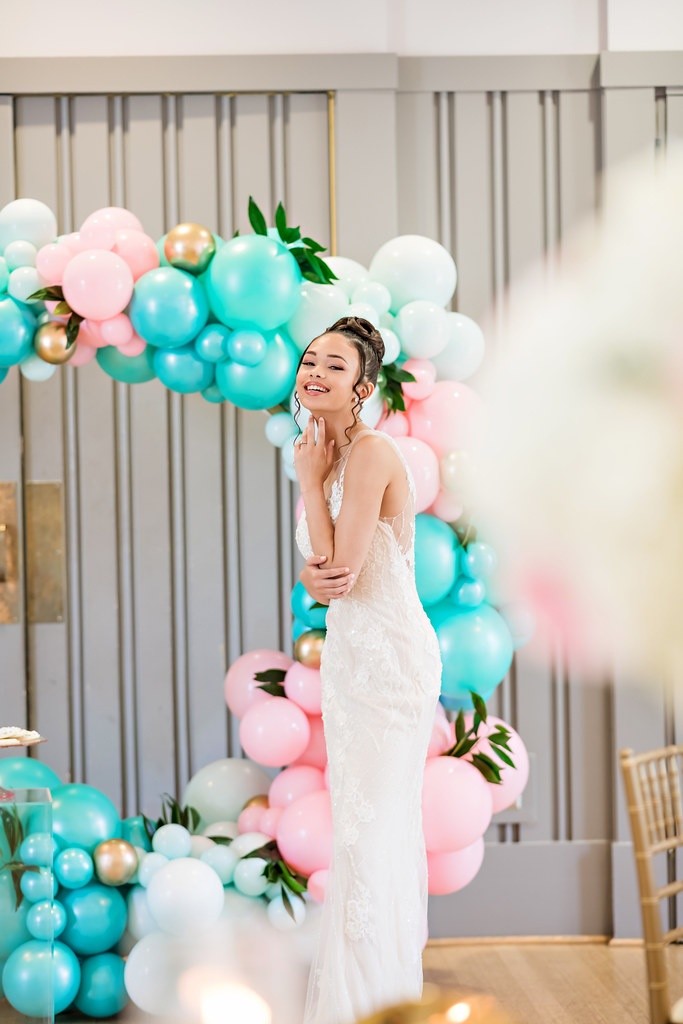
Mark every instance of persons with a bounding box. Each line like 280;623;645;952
293;318;442;1023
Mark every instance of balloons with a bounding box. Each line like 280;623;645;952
0;198;529;1024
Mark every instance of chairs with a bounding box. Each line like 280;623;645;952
619;744;682;1024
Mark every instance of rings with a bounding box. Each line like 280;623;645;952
299;441;306;449
